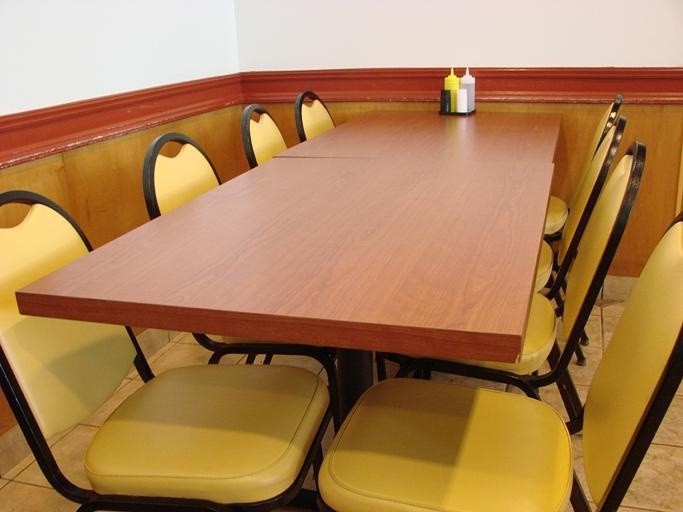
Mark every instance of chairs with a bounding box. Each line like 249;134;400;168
240;104;288;169
393;140;647;437
142;132;343;435
543;94;623;245
317;211;683;512
534;115;628;367
295;90;336;142
0;190;335;512
